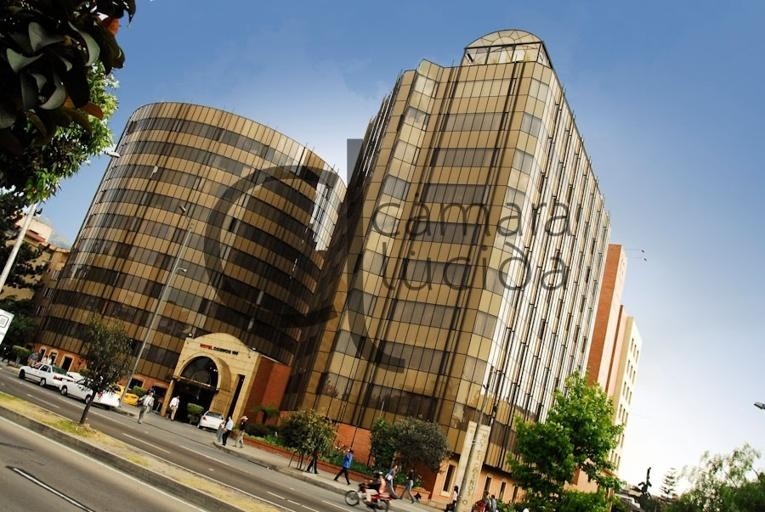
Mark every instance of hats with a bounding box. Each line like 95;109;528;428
241;416;248;420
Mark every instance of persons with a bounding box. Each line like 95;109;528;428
392;463;402;492
215;416;224;444
169;394;180;421
333;447;354;485
41;354;47;364
444;485;459;512
362;469;386;505
137;390;155;425
488;494;497;512
523;503;534;512
2;344;13;363
27;351;39;367
387;464;399;499
220;416;234;446
232;415;248;448
307;443;321;474
401;467;417;504
46;355;52;364
482;490;491;512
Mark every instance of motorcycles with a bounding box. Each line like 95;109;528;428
345;480;391;512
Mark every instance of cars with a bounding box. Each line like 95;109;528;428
58;372;121;412
16;360;75;390
195;409;226;431
112;382;140;407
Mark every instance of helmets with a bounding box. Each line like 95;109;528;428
373;470;383;477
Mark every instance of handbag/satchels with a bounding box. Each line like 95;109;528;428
385;473;392;481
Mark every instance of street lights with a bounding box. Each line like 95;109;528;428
1;142;120;292
114;201;195;404
753;401;765;410
